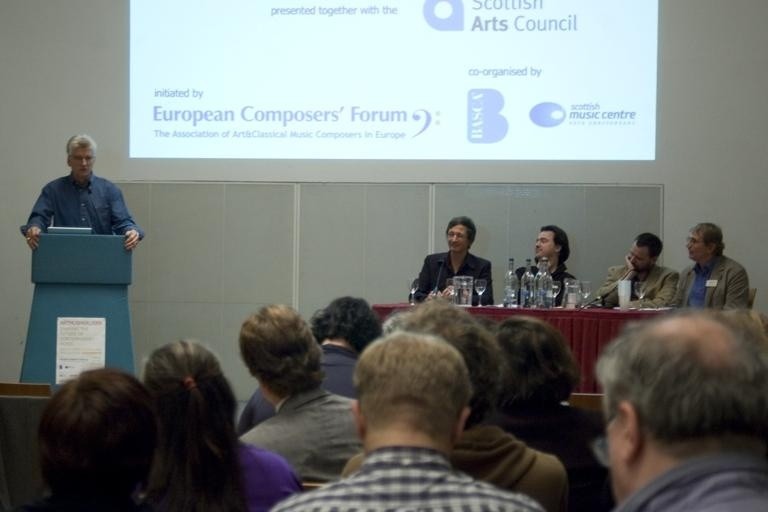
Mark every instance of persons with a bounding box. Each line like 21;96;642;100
594;307;768;511
235;304;364;493
141;341;303;512
598;233;678;309
408;216;494;306
669;223;748;311
495;315;618;512
238;296;381;436
21;133;145;250
272;328;544;511
339;298;568;512
517;225;582;307
26;368;156;511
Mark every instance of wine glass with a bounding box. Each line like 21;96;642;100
26;236;31;241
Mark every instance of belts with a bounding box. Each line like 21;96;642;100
577;280;593;308
407;277;419;305
446;278;457;303
633;282;650;309
551;281;561;307
474;280;487;308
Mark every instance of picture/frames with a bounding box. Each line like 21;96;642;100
372;304;677;394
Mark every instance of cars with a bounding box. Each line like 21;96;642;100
453;276;472;306
564;280;582;310
617;279;631;306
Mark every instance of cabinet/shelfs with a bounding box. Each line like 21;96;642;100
534;257;553;310
503;258;519;308
520;259;535;308
